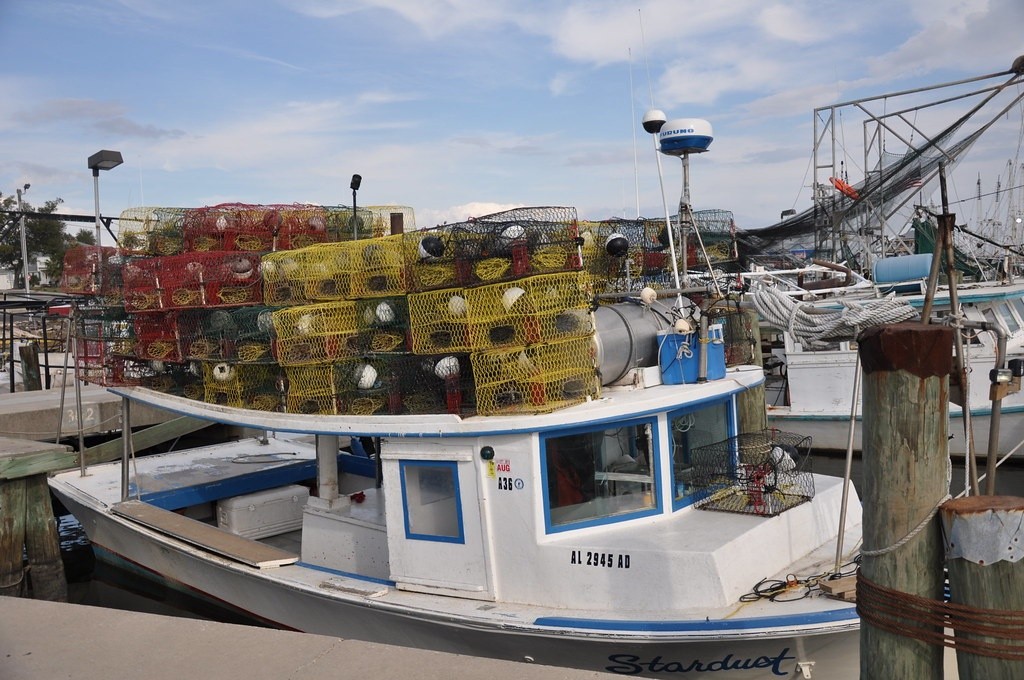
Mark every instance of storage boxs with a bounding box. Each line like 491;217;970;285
62;204;600;418
574;210;738;307
216;484;309;541
688;430;814;517
657;324;726;384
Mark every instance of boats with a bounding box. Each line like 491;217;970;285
0;289;155;390
686;264;873;300
764;283;1024;457
45;108;861;679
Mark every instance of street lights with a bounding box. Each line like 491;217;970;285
87;150;125;291
